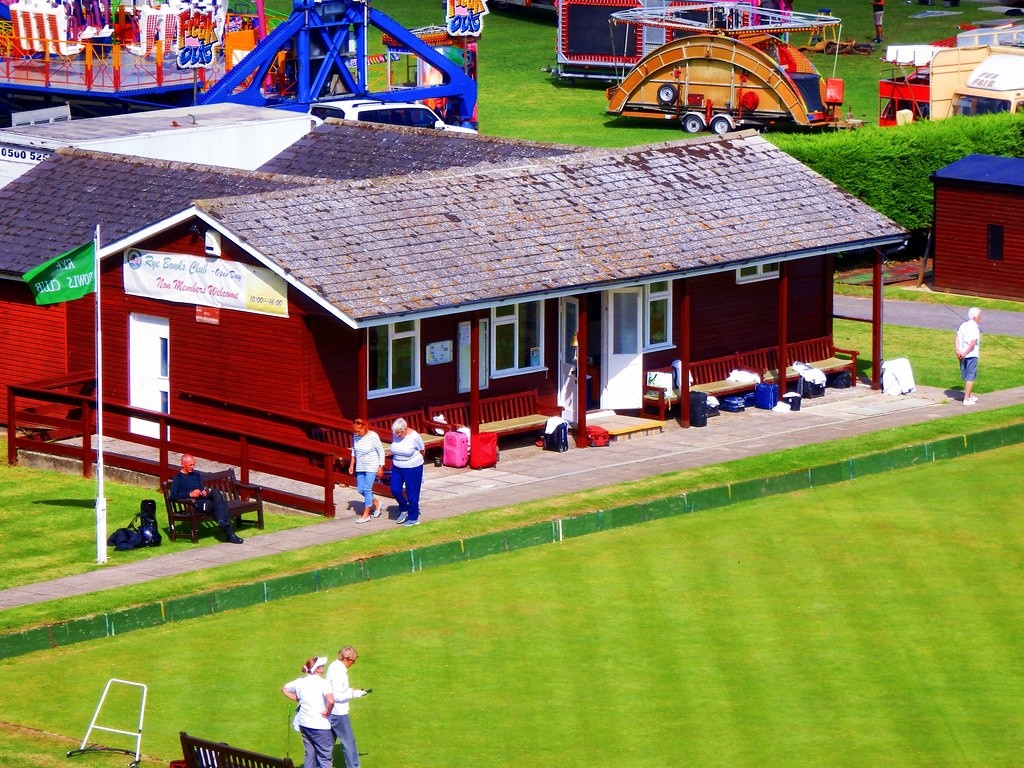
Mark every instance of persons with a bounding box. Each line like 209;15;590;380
390;417;426;527
281;656;335;768
324;645;369;768
954;307;982;406
348;419;385;523
170;454;244;543
869;0;885;43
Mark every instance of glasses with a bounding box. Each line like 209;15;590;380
353;421;365;426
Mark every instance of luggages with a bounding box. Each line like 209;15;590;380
544;419;569;453
783;396;801;410
690;383;779;427
443;431;468;468
797;376;825;399
469;432;497;471
585;425;610;447
826;371;851;389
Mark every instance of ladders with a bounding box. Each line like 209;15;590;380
807;8;836;48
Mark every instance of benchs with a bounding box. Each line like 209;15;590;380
179;731;294;768
161;467;264;543
311;389;566;476
640;336;859;421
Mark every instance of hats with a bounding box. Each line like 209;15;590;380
305;657;328;675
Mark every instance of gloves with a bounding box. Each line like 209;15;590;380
352;690;367;698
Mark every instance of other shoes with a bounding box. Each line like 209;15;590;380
220;522;231;531
227;534;243;543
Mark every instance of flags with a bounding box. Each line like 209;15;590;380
24;239;97;304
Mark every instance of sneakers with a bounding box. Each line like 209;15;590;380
356;516;371;523
402;517;420;527
374;501;382;518
969;396;978;401
962;399;975;406
396;511;408;523
